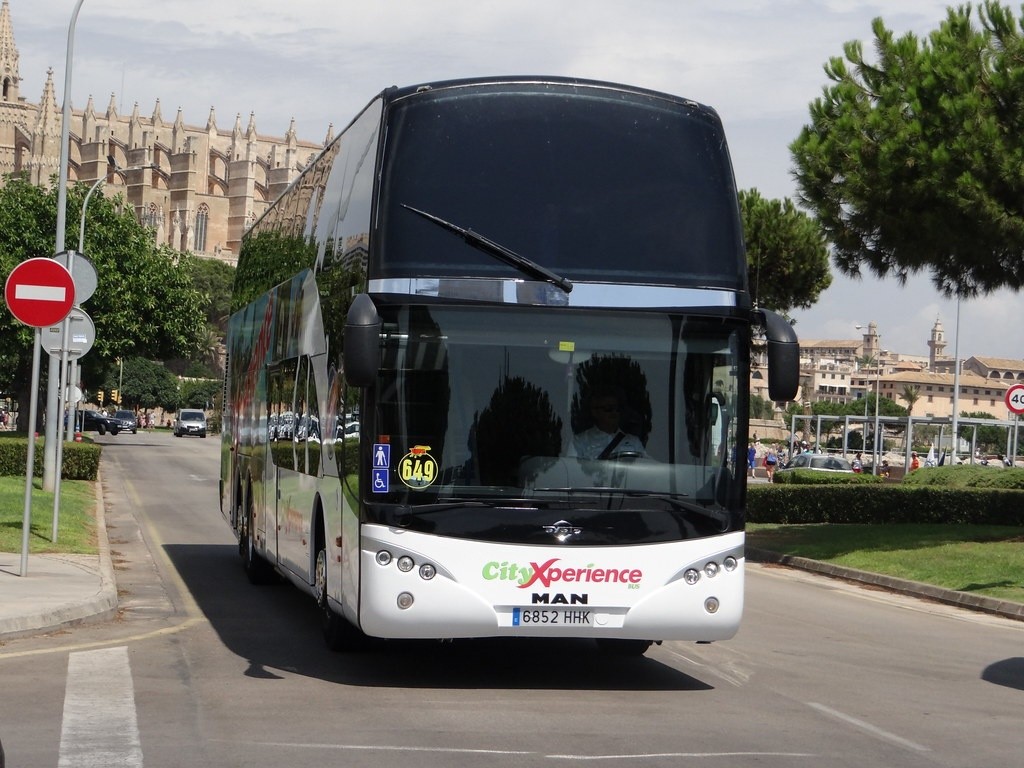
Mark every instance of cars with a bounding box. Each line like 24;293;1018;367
772;453;855;473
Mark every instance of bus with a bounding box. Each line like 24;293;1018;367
217;71;801;640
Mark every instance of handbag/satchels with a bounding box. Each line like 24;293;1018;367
763;456;767;466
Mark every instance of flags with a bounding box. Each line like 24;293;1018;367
922;442;946;467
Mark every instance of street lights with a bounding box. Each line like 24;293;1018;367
862;349;887;455
855;325;880;478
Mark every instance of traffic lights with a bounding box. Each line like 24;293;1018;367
98;391;104;402
117;396;122;405
111;390;118;402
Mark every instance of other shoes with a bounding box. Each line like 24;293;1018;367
753;476;756;479
768;478;771;482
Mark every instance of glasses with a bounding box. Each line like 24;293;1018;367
592;404;624;412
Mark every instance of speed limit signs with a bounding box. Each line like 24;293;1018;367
1004;384;1024;414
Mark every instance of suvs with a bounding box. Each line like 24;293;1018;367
114;410;138;434
64;408;123;436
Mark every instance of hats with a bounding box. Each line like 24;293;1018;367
802;441;807;444
912;452;917;455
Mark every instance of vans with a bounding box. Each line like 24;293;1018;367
172;408;207;438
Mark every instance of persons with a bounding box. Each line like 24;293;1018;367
0;411;13;430
998;454;1011;467
101;405;171;431
718;426;892;480
563;389;656;461
909;452;919;471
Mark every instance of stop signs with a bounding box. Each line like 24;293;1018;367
3;258;75;328
